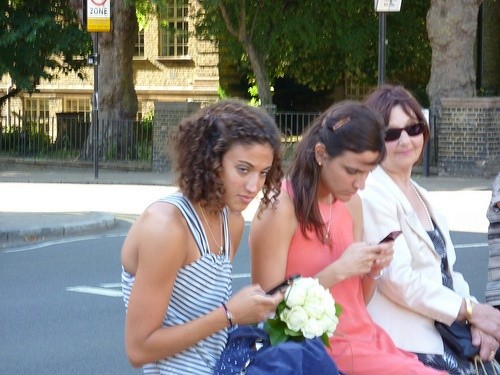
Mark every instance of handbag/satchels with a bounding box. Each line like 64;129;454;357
213;325;271;375
434;319;481;361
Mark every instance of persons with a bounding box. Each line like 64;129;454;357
120;100;285;375
485;173;500;364
356;84;500;375
249;101;448;375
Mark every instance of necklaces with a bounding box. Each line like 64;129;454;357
410;183;434;230
199;201;224;255
320;193;332;239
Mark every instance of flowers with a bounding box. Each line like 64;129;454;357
264;276;342;353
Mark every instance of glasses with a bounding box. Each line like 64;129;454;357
383;122;426;142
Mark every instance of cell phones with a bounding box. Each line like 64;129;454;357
266;274;302;295
379;230;402;244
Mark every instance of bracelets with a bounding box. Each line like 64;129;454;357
368;270;382;280
224;305;232;327
465;298;473;318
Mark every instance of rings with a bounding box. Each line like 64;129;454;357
491;351;495;357
